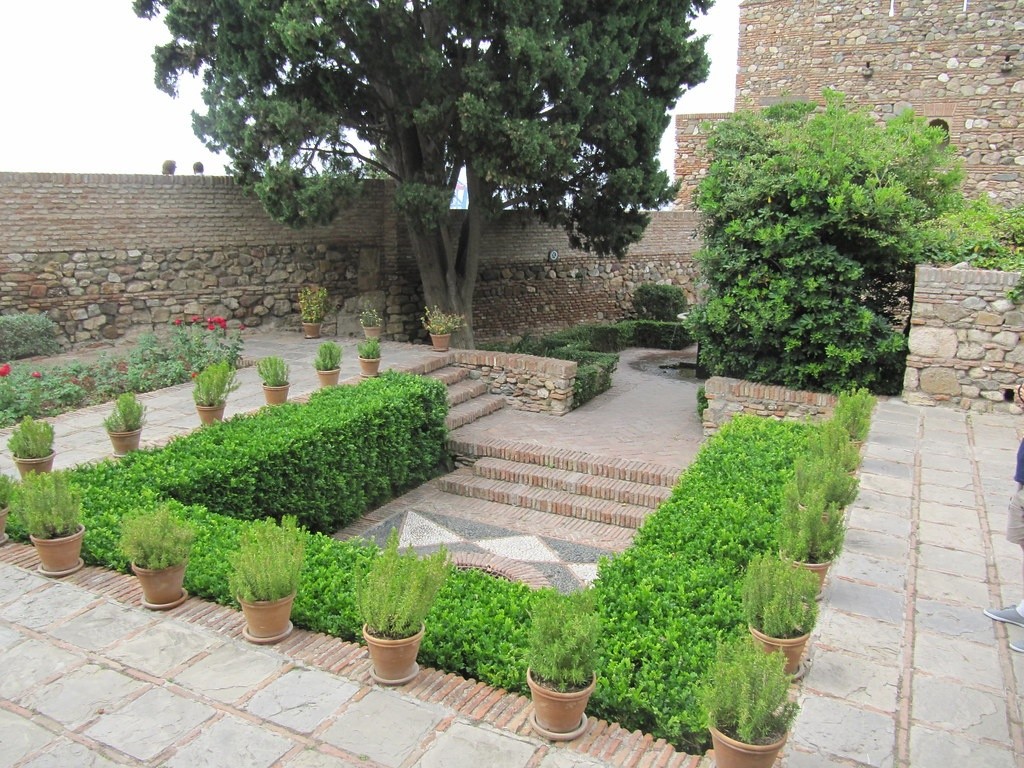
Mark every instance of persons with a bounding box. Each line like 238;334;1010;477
162;160;176;176
984;434;1024;652
193;161;204;176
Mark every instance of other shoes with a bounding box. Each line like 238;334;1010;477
1009;640;1024;653
984;604;1024;628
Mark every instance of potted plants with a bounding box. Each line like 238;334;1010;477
704;382;876;768
191;360;241;426
312;341;343;387
120;506;191;604
8;415;56;478
19;471;85;572
226;514;306;639
257;357;291;405
526;598;602;732
298;287;329;338
354;526;455;680
358;337;381;375
0;475;13;539
103;392;145;455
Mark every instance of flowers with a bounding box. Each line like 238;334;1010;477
359;308;383;327
419;305;465;335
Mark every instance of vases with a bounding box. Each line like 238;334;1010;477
430;333;451;351
363;326;382;341
